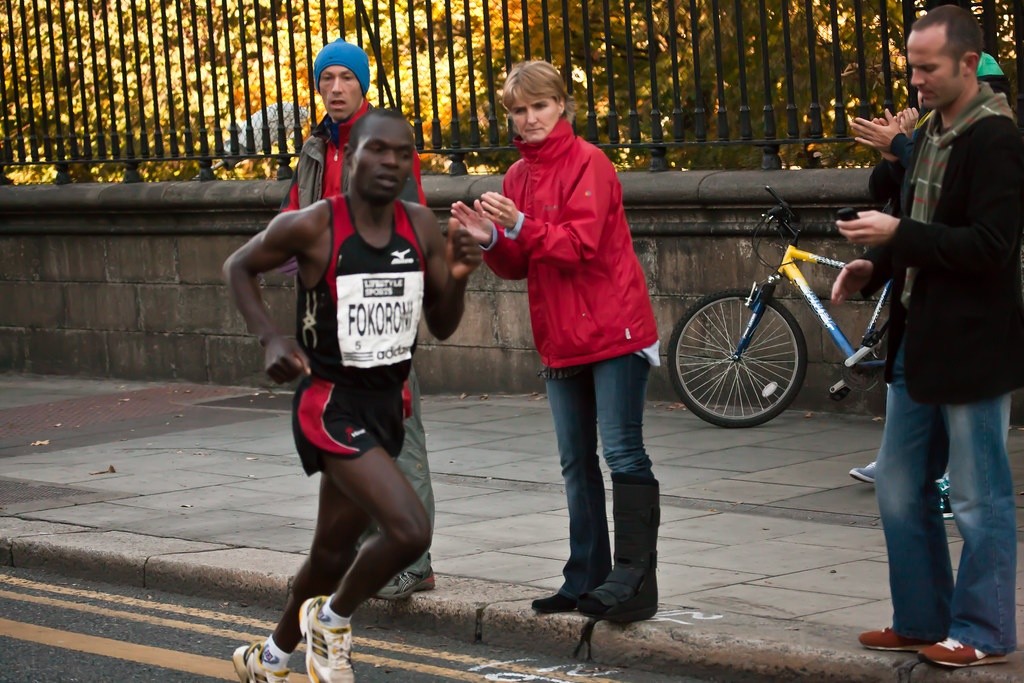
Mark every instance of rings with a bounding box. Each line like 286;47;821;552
499;212;503;217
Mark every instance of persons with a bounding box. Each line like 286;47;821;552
451;61;661;620
832;5;1024;669
280;38;436;601
222;111;483;683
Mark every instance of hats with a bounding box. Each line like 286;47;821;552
313;38;370;99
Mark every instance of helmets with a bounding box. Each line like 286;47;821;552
976;52;1004;77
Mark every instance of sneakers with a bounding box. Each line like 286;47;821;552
372;566;435;600
233;640;291;683
934;472;955;519
858;627;934;651
298;595;355;683
917;637;1008;667
849;461;876;483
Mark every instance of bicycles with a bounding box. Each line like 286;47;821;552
667;186;895;429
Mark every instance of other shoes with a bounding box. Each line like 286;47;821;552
531;594;579;613
577;590;658;623
